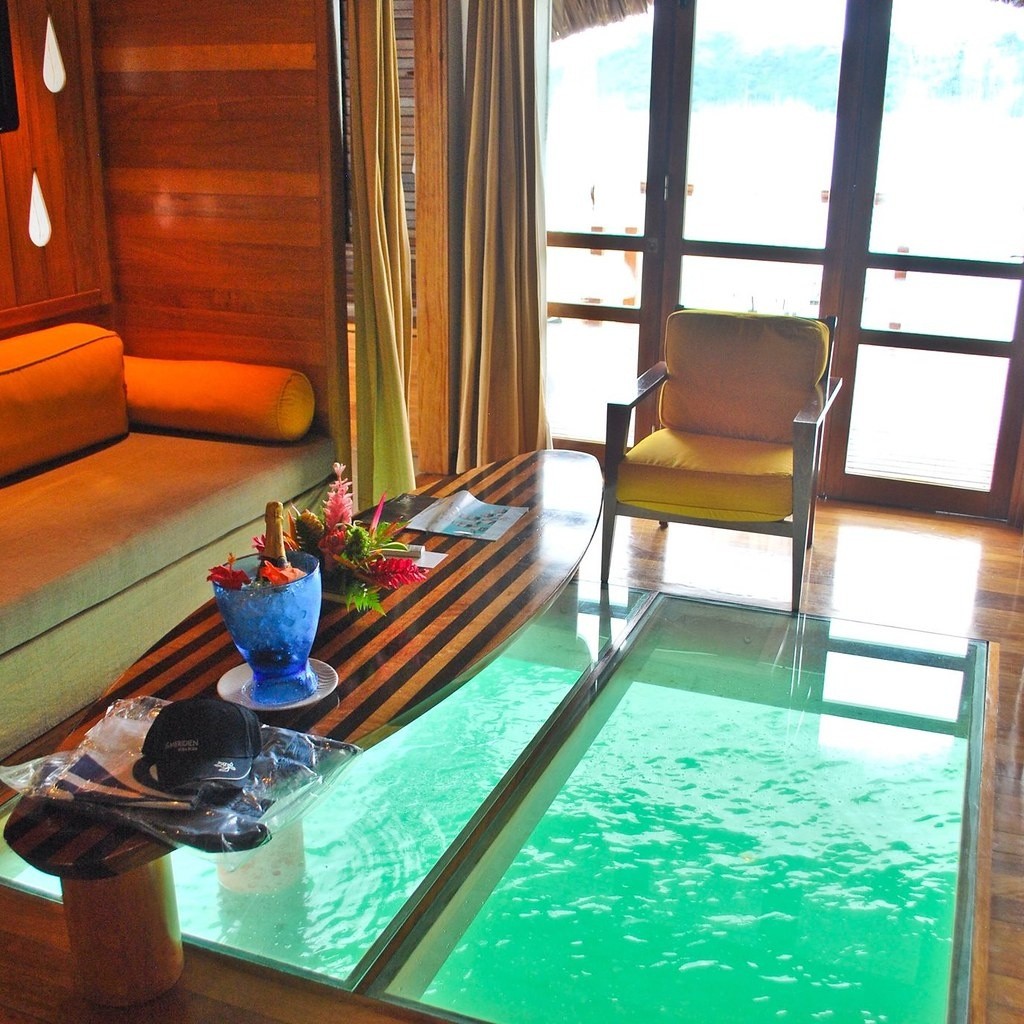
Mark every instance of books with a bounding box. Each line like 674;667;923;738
354;490;530;543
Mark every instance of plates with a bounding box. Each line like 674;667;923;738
217;656;338;711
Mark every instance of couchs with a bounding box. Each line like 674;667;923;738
0;321;338;761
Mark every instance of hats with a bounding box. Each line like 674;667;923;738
140;697;262;788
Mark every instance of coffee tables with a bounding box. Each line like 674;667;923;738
4;448;604;1007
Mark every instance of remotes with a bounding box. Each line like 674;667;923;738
381;545;426;557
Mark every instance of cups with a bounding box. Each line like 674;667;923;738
213;548;320;706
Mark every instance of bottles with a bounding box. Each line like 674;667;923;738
256;501;292;582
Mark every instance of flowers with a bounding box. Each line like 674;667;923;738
249;463;428;617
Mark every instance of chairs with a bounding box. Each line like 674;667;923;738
601;304;843;611
